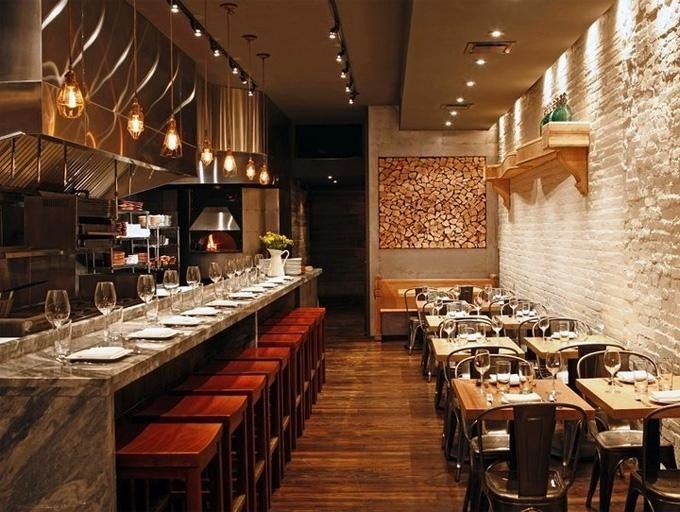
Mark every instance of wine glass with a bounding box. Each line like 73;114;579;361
433;299;442;317
538;315;550;345
137;273;156;323
185;264;201;307
491;315;504;343
421;286;430;303
208;262;222;296
473;349;491;395
603;348;622;394
232;256;245;284
443;319;454;343
44;288;73;364
656;356;674;391
545;350;562;400
242;255;254;286
632;359;649;401
484;284;492;304
224;258;236;289
161;269;180;310
93;281;117;340
473;295;483;315
509;296;518;320
518;361;534;395
452;285;462;302
256;253;267;279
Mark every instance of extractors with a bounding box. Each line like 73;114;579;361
188;206;240;231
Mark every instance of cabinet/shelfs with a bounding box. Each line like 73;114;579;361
108;210;151;275
131;225;180;277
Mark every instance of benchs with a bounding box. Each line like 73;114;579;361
373;274;500;343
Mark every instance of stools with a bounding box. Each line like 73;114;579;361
260;325;310;431
210;347;292;479
163;374;270;511
584;430;678;512
272;312;321;400
265;317;316;414
120;394;250;512
188;359;281;488
462;434;510;512
249;333;304;450
113;421;225;512
280;306;326;384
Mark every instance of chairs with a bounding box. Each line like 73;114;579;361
403;287;439;351
446;284;474;304
487;299;530;318
436;317;499;338
446;346;520;379
500;301;548;316
477;288;515;298
408;290;459;356
557;344;625;361
437;319;494;340
532;317;593;379
454;353;536;382
461;401;588;512
517;318;541;348
624;401;680;512
570;350;659;485
419;299;467;368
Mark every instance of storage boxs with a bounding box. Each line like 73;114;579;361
23;191;79;250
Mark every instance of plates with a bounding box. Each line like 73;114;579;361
183;306;224;316
227;289;254;299
257;281;277;288
614;368;656;384
499;391;543;405
160;314;202;327
241;284;266;294
206;297;244;308
487;373;524;387
139;214;171;227
266;277;285;284
125;325;179;339
648;389;680;404
276;272;294;279
62;346;135;361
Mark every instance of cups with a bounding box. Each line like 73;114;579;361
474;322;487;343
146;295;159;321
193;284;204;306
171;289;182;311
558;321;570;338
107;306;124;339
457;322;468;344
495;360;511;394
522;302;529;317
446;303;457;317
51;319;72;354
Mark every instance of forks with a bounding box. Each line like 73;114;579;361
484;391;495;408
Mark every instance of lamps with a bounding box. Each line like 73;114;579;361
256;52;271;186
159;1;183;159
188;206;242;253
54;0;85;121
219;4;239;179
238;34;257;182
197;0;214;167
125;1;145;141
